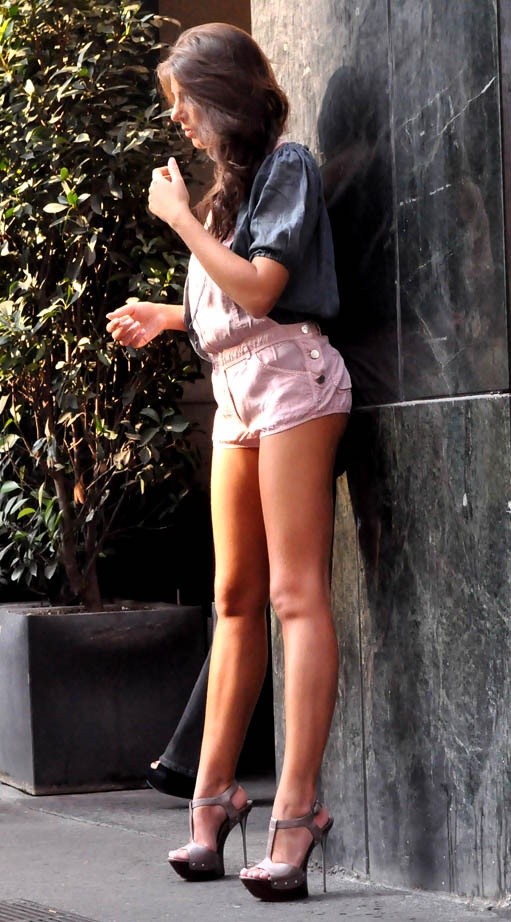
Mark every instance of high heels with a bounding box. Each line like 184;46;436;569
168;782;255;882
240;796;334;901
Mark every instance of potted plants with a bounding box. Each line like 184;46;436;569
0;0;217;798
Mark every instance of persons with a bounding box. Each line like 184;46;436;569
107;20;351;902
